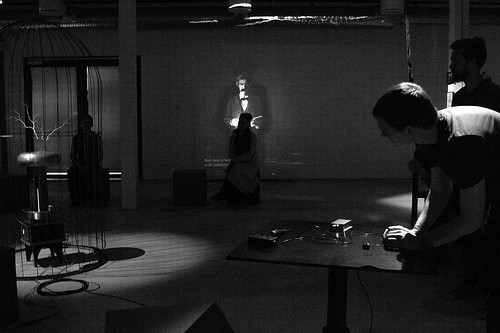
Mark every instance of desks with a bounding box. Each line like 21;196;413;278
226;219;411;333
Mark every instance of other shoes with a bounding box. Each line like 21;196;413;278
442;282;480;301
210;191;225;201
476;309;486;319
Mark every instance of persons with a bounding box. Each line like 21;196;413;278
209;113;259;205
372;81;500;303
450;36;500;112
65;114;105;209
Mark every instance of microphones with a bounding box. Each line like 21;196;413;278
271;229;292;236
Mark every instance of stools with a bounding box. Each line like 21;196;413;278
173;169;206;205
227;168;260;205
92;168;110;203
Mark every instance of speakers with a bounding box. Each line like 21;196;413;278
106;299;235;333
